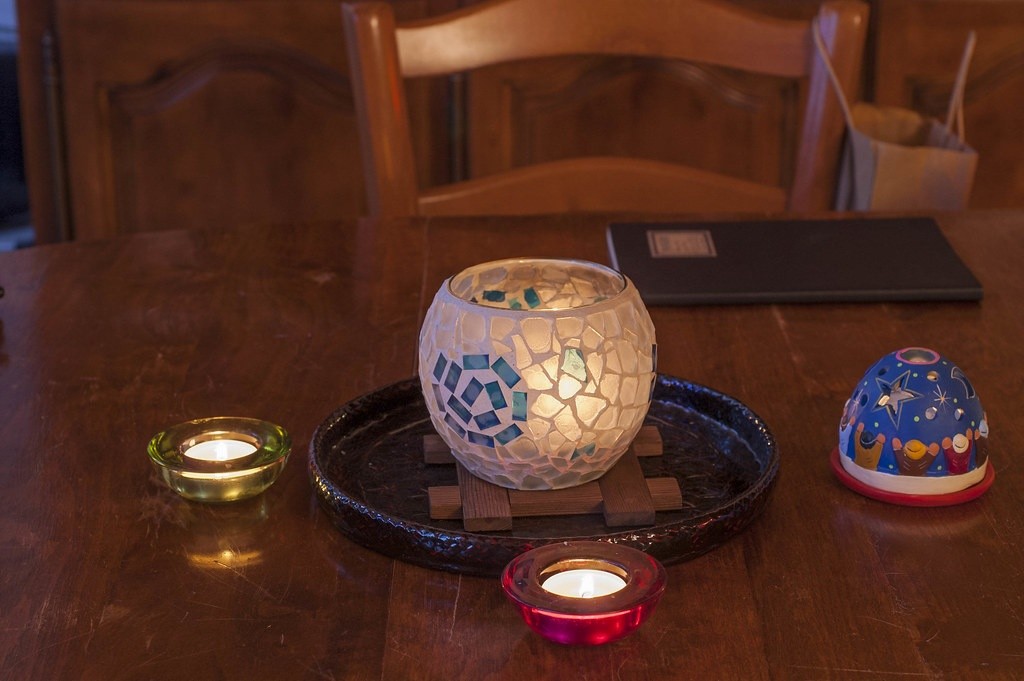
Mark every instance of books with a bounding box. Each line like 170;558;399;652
604;216;983;308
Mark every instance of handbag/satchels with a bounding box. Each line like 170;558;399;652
812;19;979;212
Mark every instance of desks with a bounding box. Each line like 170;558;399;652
0;212;1022;681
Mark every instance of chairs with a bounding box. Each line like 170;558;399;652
344;0;871;211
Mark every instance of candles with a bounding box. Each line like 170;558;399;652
149;415;287;500
415;256;660;493
500;538;668;646
